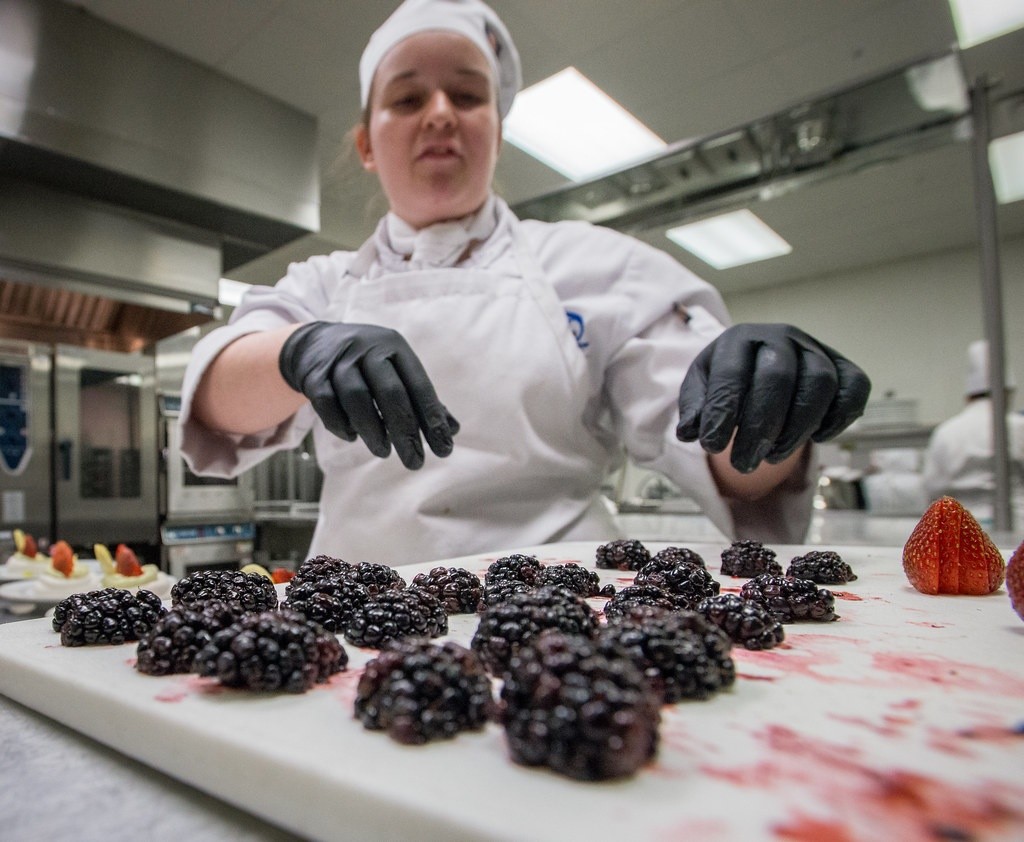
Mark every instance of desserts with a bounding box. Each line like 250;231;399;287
3;529;296;602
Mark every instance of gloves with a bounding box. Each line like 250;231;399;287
279;319;460;469
676;324;873;474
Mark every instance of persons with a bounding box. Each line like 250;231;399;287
176;0;867;568
923;338;1023;550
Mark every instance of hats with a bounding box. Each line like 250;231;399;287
358;0;523;123
962;341;1016;397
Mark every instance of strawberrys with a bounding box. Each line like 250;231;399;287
1005;538;1024;624
902;496;1007;595
